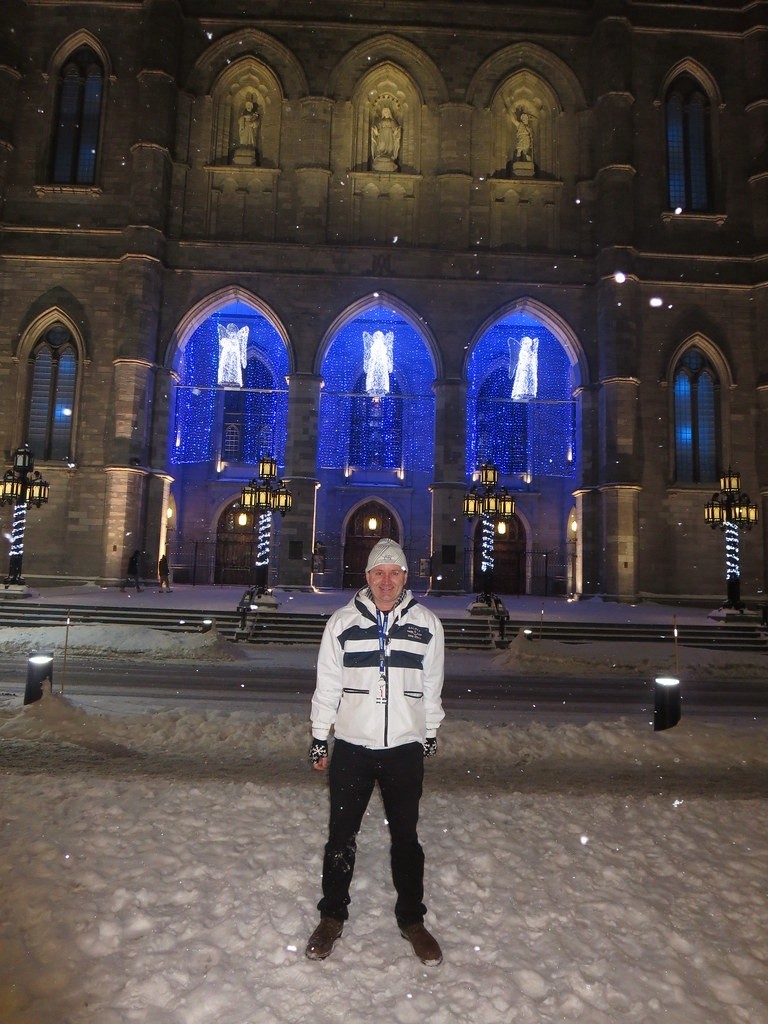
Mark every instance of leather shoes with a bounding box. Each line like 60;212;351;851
306;917;344;960
401;921;442;967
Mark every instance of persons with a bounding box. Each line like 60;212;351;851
158;555;173;593
120;549;144;593
508;109;533;161
305;537;446;967
371;107;402;162
238;101;261;148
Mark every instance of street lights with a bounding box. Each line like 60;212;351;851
703;463;759;607
241;450;293;594
463;455;516;593
0;442;51;586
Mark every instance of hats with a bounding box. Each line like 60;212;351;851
365;538;408;573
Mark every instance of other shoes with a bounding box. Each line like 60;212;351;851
159;591;164;593
120;590;127;592
137;589;144;592
167;591;173;593
